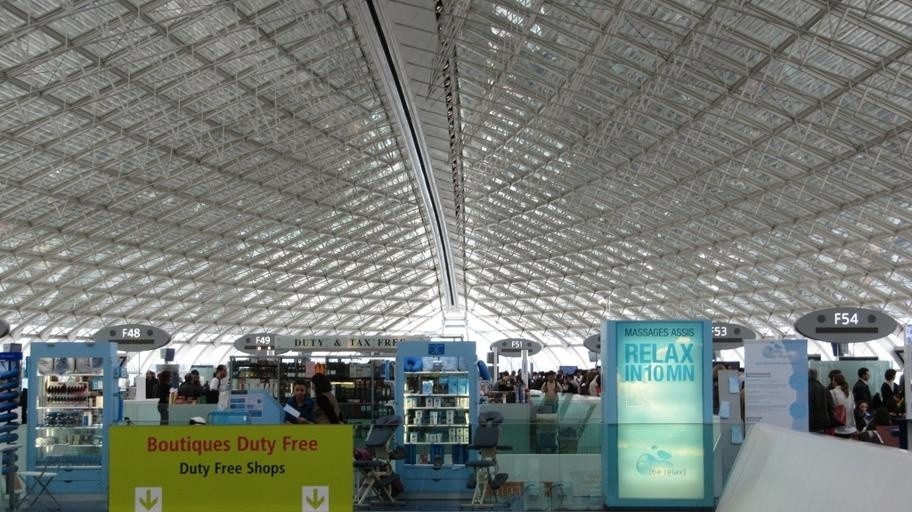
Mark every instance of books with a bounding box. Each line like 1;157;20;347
283;403;302;420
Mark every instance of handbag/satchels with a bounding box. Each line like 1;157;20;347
206;377;220;403
833;404;847;426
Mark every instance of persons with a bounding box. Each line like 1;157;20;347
283;380;317;425
297;372;344;427
142;364;228;427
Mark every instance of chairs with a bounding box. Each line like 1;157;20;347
353;415;406;509
455;411;510;511
14;442;65;512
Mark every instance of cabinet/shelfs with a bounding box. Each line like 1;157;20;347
30;340;118;494
227;355;396;426
395;341;480;495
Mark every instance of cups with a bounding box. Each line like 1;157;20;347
403;375;469;466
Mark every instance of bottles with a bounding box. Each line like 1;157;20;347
232;360;394;417
